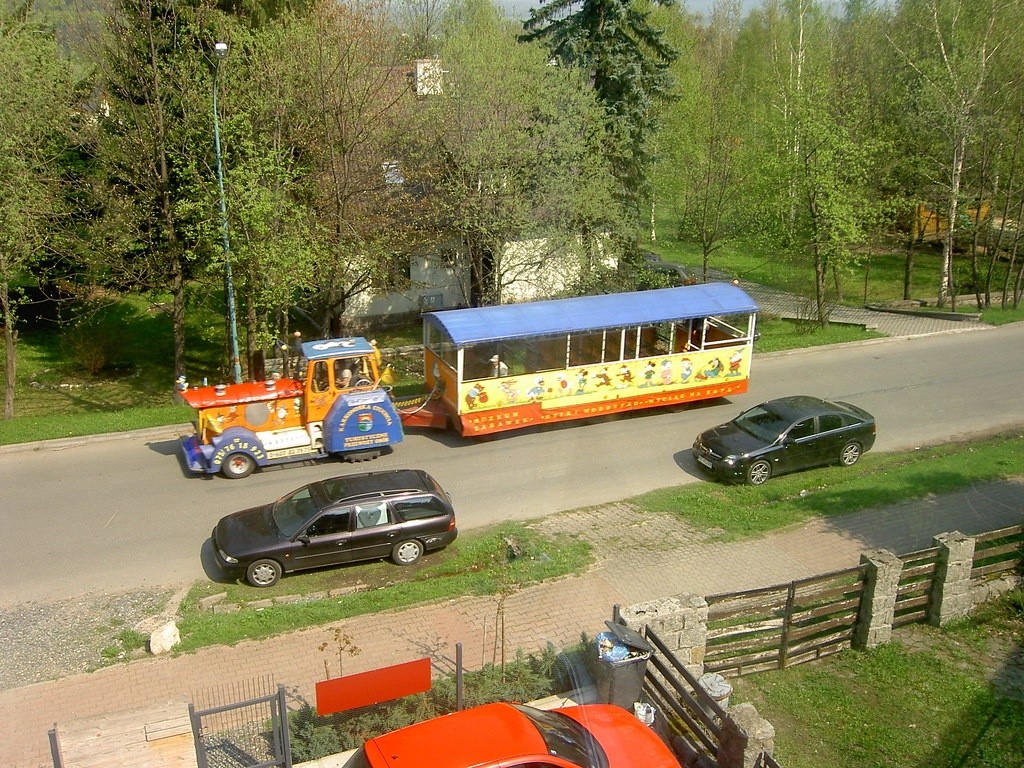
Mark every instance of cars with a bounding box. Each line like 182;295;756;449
342;700;683;768
691;395;877;486
210;469;458;588
619;247;698;287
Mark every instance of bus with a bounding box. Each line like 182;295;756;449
176;281;763;479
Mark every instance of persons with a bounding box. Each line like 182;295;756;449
335;369;352;388
489;355;508;376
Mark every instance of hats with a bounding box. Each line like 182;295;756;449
490;355;498;362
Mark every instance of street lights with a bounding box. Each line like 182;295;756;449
211;42;245;385
